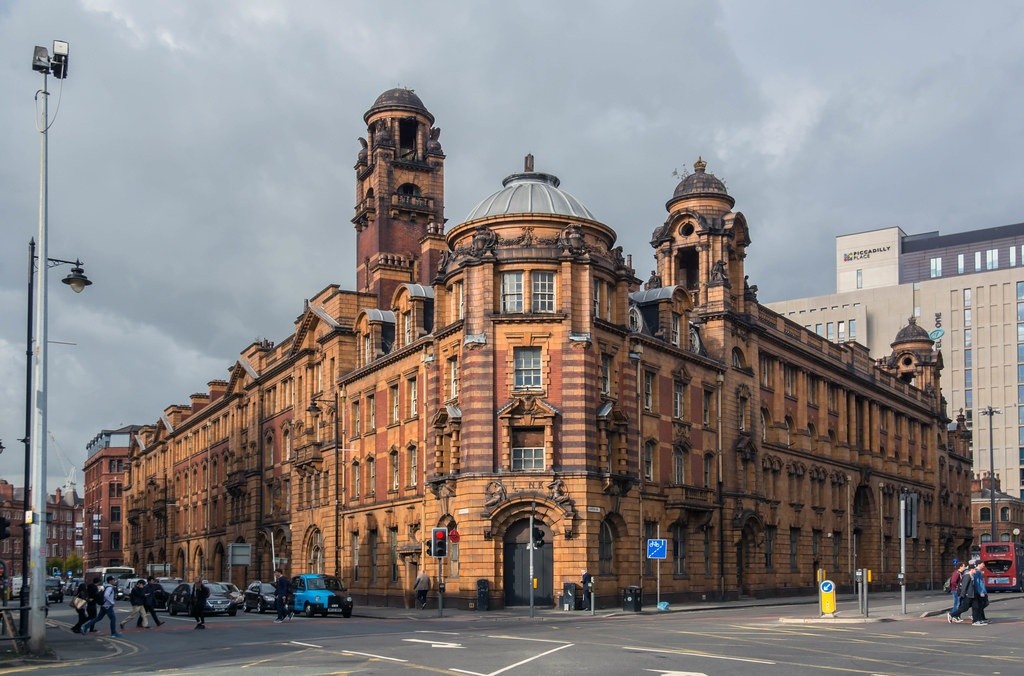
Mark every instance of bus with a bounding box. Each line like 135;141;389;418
979;542;1024;593
84;567;134;596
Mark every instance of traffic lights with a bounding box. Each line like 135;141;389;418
432;528;448;557
533;527;545;547
426;540;433;556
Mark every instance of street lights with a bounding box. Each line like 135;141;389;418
85;506;100;564
307;390;340;578
147;472;167;577
982;406;1003;542
29;37;70;649
19;237;94;652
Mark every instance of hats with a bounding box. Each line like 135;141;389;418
107;576;116;581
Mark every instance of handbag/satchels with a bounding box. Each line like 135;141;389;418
72;594;89;610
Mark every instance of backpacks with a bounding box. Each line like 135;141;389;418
95;585;113;606
943;576;952;594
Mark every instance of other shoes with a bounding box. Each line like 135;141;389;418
70;627;81;633
952;616;963;624
89;629;97;632
81;626;86;635
120;623;124;629
111;633;122;638
421;603;427;610
969;616;992;626
274;612;294;624
194;622;204;629
584;608;591;611
157;622;165;626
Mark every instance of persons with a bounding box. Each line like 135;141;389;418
72;576;122;638
274;569;294;623
948;560;992;625
191;576;206;629
120;576;165;628
580;570;591;610
413;570;431;609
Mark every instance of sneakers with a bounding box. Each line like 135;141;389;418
948;612;952;624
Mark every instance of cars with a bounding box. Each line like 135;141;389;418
168;580;238;618
289;574;354;619
7;576;83;604
213;581;245;609
113;577;183;611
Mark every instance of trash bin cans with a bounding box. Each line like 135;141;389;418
623;586;642;611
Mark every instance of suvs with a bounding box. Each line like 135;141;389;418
242;581;294;615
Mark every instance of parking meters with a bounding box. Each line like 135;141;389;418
438;582;445;616
855;569;864;614
588;582;595;615
